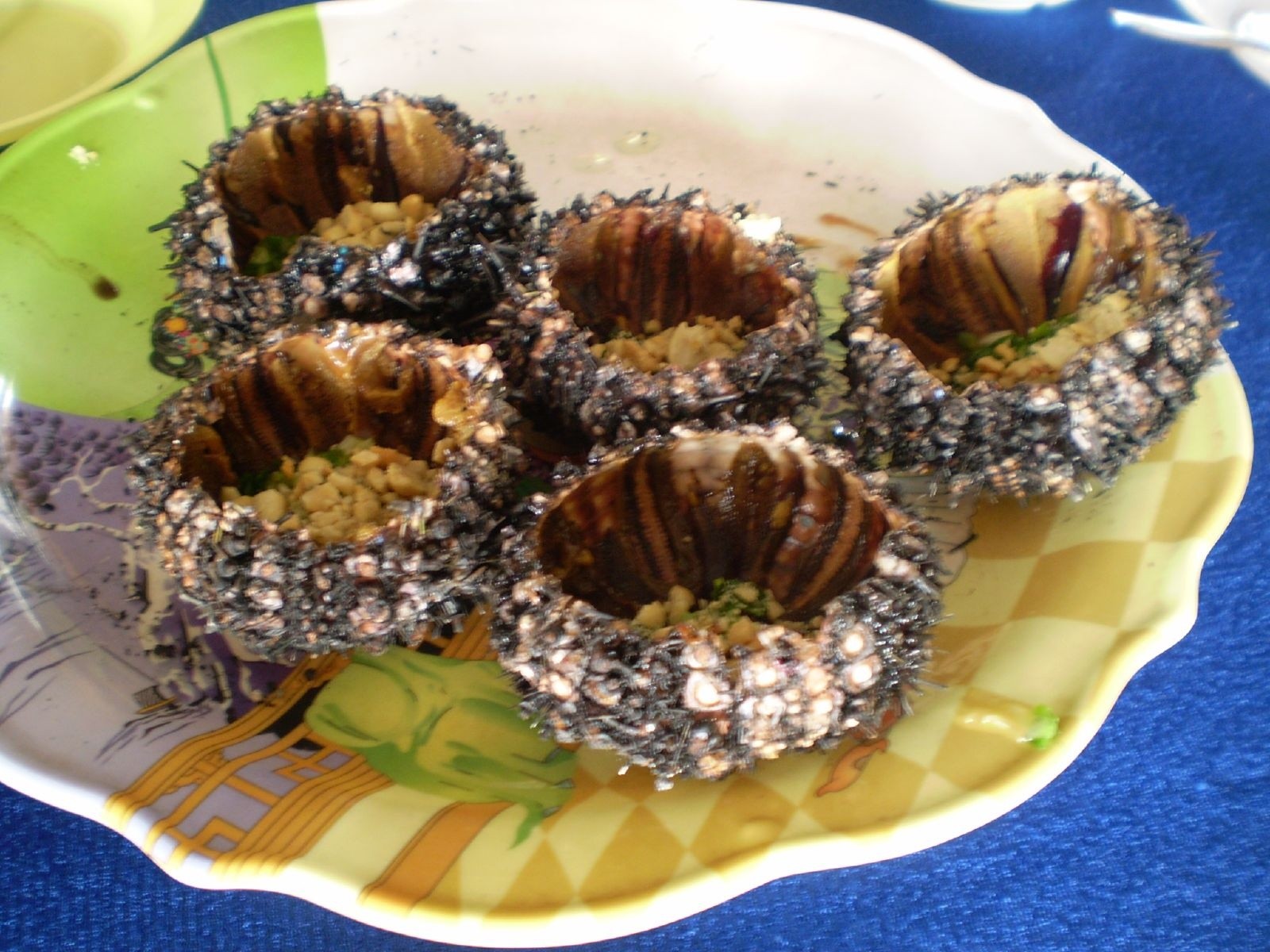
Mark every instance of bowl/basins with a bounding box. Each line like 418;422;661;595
0;0;204;147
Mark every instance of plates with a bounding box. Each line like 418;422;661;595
0;0;1255;948
